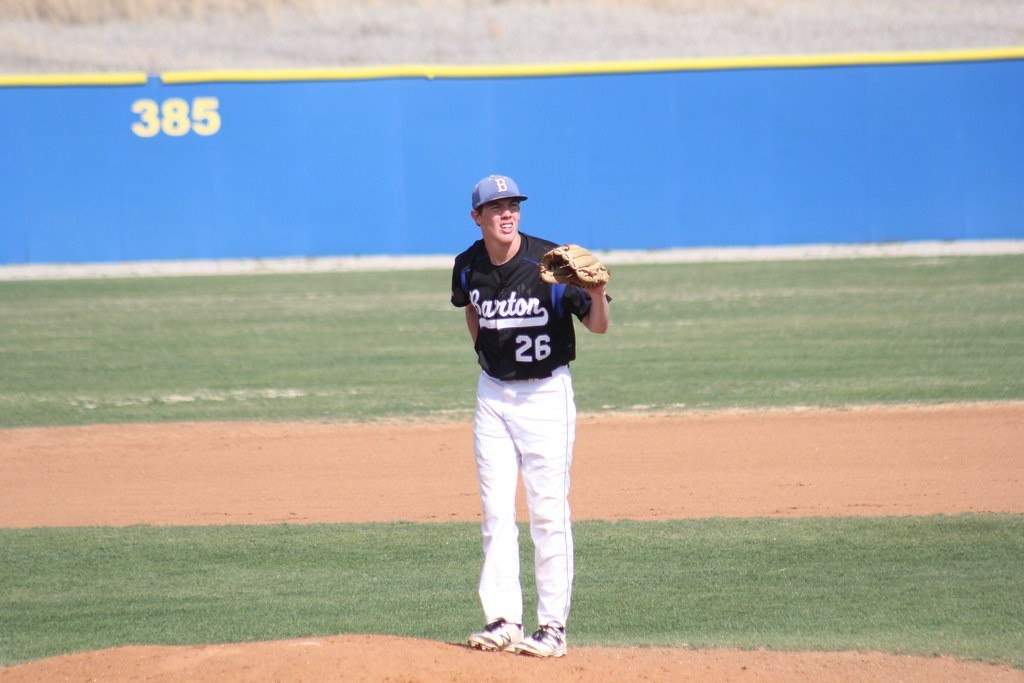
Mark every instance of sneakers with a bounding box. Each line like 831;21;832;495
468;619;524;652
514;626;568;658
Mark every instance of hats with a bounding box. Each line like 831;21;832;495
472;174;528;210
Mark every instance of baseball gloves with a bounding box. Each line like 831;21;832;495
539;244;611;287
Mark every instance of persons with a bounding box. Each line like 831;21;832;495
452;173;613;659
